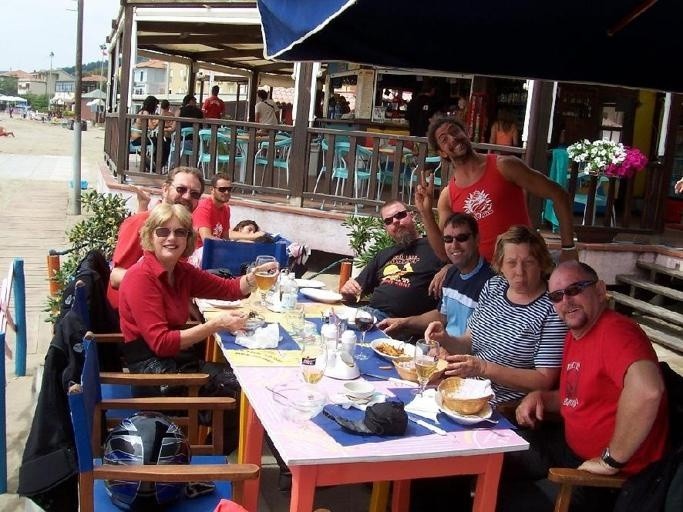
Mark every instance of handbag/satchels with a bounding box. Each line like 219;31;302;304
363;401;408;436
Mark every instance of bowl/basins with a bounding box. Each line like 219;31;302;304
243;314;264;331
343;381;374;405
371;337;421;361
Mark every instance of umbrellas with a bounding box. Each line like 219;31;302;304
252;0;683;94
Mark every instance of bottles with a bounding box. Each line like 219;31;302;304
277;269;316;343
319;324;356;359
328;101;340;120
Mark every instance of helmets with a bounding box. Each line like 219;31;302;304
103;411;191;512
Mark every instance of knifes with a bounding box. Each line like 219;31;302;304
406;412;446;435
365;373;418;387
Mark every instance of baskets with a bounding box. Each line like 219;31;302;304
438;376;489;415
392;355;449;384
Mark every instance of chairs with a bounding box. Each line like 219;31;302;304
56;300;207;444
278;237;310;279
127;126;618;235
200;241;287;277
67;330;261;512
73;249;208;369
494;361;683;512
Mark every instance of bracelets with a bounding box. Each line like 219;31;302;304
245;276;255;287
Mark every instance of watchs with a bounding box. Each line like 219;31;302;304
601;448;625;468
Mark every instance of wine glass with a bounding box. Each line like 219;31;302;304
410;340;439;396
255;257;277;321
354;306;374;361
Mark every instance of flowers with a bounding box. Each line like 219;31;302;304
566;138;649;179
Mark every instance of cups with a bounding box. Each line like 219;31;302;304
300;340;326;386
326;313;348;342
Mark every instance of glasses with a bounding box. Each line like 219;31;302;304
176;187;201;199
547;280;595;303
215;187;232;192
153;228;187;237
441;233;473;242
384;210;409;225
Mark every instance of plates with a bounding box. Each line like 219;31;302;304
211;300;241;307
272;382;328;410
294;278;325;288
337;304;372;328
300;288;343;305
435;389;491;424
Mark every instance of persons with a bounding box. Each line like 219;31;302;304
133;86;225;146
8;104;13;118
106;86;293;493
119;203;277;456
395;92;517;155
106;166;204;311
415;116;579;269
188;173;232;266
228;220;266;242
423;226;569;404
408;260;666;512
340;82;671;512
338;201;495;337
327;88;350;119
22;107;27;118
251;90;293;135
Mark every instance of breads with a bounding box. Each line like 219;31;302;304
375;343;398;355
